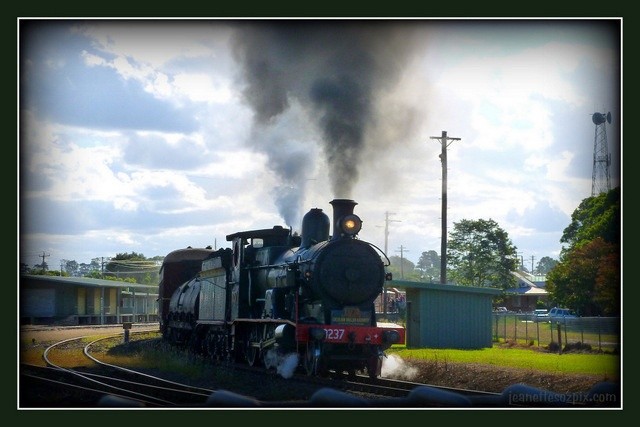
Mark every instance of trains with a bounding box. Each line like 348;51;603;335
157;197;408;382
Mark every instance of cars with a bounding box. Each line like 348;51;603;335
530;309;552;322
548;306;581;320
